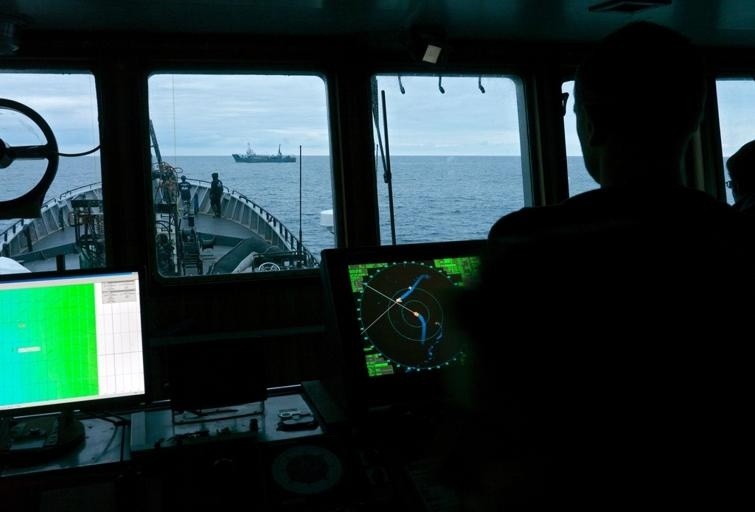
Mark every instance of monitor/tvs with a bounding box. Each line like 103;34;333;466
319;239;488;408
146;266;334;414
0;262;153;415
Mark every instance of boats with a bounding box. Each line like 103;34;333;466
232;143;296;162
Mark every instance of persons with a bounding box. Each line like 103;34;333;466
179;176;191;213
210;174;222;218
458;22;755;512
727;142;755;216
